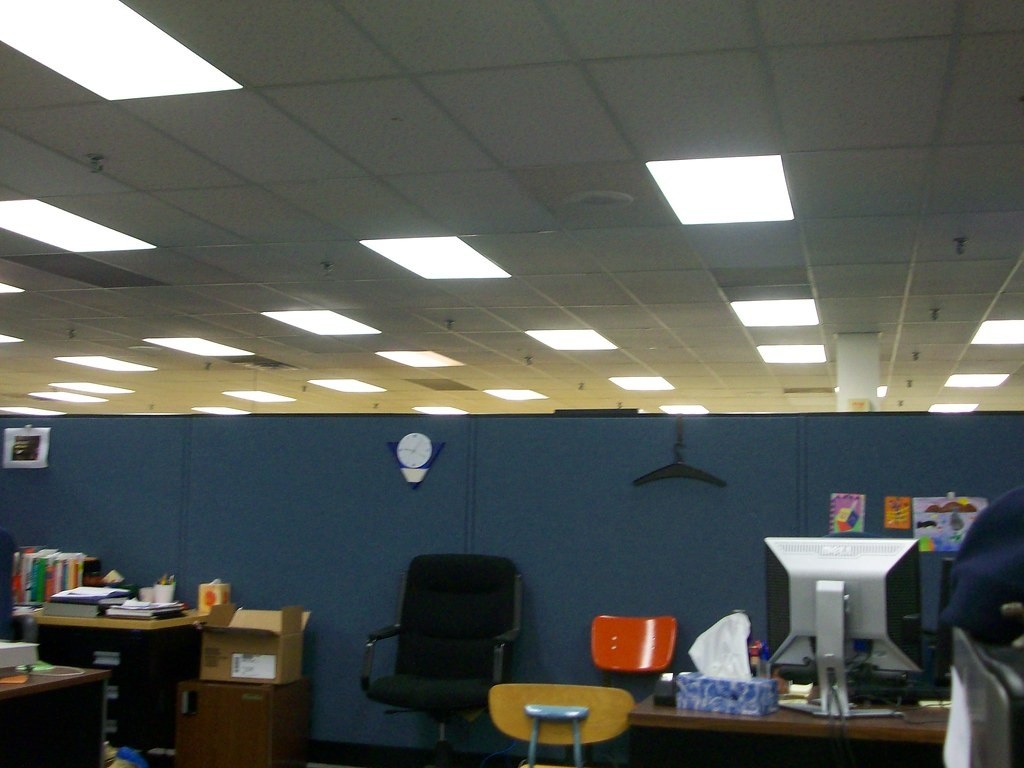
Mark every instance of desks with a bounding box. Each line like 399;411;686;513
627;666;953;768
32;607;219;758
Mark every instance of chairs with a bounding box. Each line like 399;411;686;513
587;615;677;768
360;553;524;768
488;683;635;768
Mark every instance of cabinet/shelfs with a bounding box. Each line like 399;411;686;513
174;679;313;768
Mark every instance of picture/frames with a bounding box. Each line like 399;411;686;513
4;426;51;469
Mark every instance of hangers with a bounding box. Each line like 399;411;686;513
632;441;730;490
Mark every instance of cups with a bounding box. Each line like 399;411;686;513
139;588;155;602
154;583;174;605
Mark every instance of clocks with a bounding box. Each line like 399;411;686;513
396;431;433;468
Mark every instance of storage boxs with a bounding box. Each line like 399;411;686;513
0;665;110;768
200;604;312;686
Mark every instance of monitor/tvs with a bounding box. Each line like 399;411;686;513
764;536;925;718
936;558;955;687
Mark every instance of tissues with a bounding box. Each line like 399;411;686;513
674;611;780;718
197;578;231;613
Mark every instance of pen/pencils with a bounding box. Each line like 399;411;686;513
159;572;176;586
